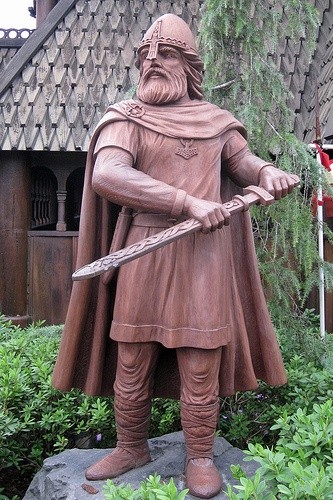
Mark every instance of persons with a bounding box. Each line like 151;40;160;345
48;13;290;498
